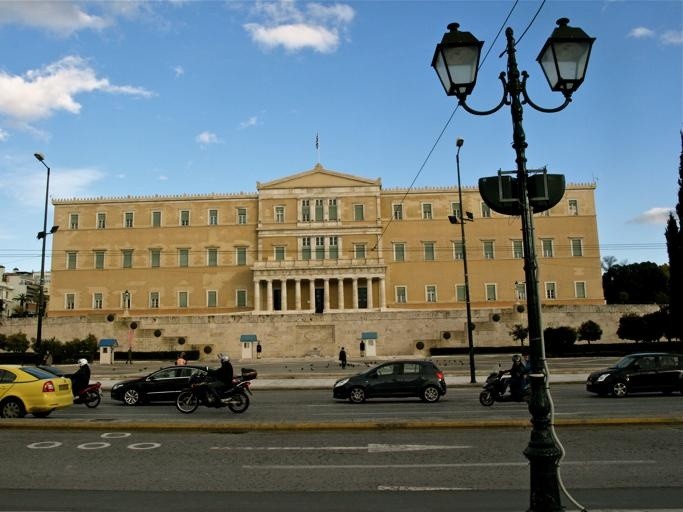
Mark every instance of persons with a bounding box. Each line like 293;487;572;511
43;348;53;367
199;354;233;406
175;352;187;366
62;357;90;402
521;351;532;392
125;346;132;364
359;339;365;357
338;347;346;369
500;353;523;392
255;341;262;359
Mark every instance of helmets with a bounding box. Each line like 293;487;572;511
512;355;521;363
220;355;230;364
78;359;88;366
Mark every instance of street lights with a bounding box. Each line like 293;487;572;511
122;289;130;316
31;151;59;361
432;16;596;512
451;136;477;383
512;279;521;303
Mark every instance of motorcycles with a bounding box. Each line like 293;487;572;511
479;364;530;406
73;381;101;408
176;365;256;413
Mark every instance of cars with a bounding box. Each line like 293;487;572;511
36;366;74;380
585;352;682;397
110;365;216;405
0;364;73;417
332;358;446;404
